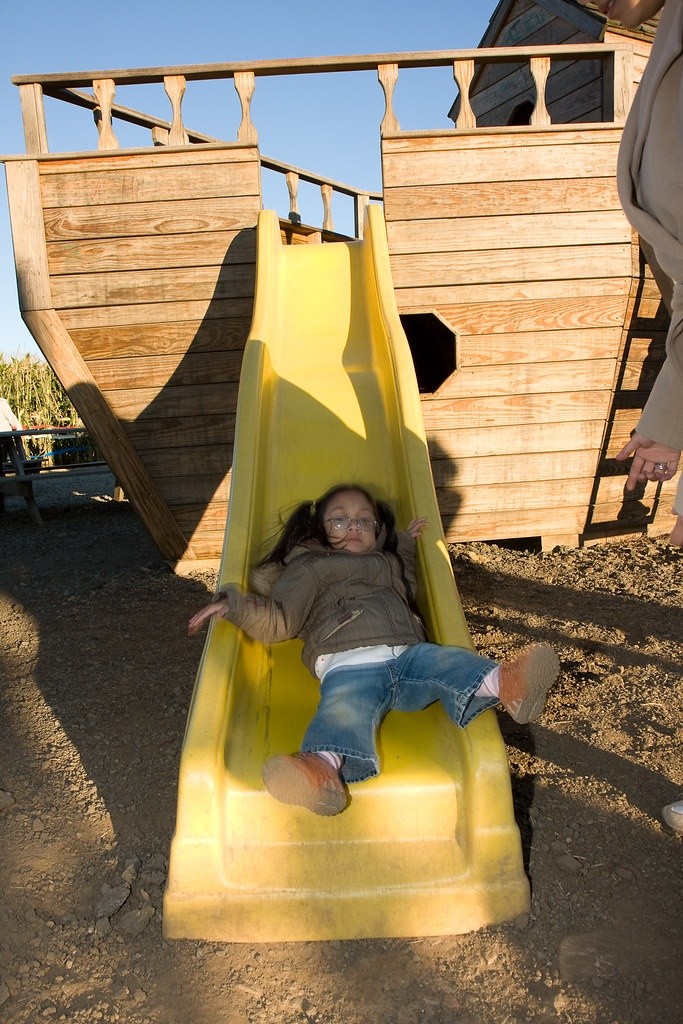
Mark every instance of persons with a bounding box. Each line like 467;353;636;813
0;398;22;477
568;0;683;837
188;485;560;817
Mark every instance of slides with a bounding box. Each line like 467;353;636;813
160;204;532;943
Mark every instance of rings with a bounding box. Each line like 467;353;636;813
654;464;667;470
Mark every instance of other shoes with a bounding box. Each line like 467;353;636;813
662;800;682;832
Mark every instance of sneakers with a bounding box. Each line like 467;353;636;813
498;645;560;724
262;751;348;816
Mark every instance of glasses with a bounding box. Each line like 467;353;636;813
323;515;380;532
